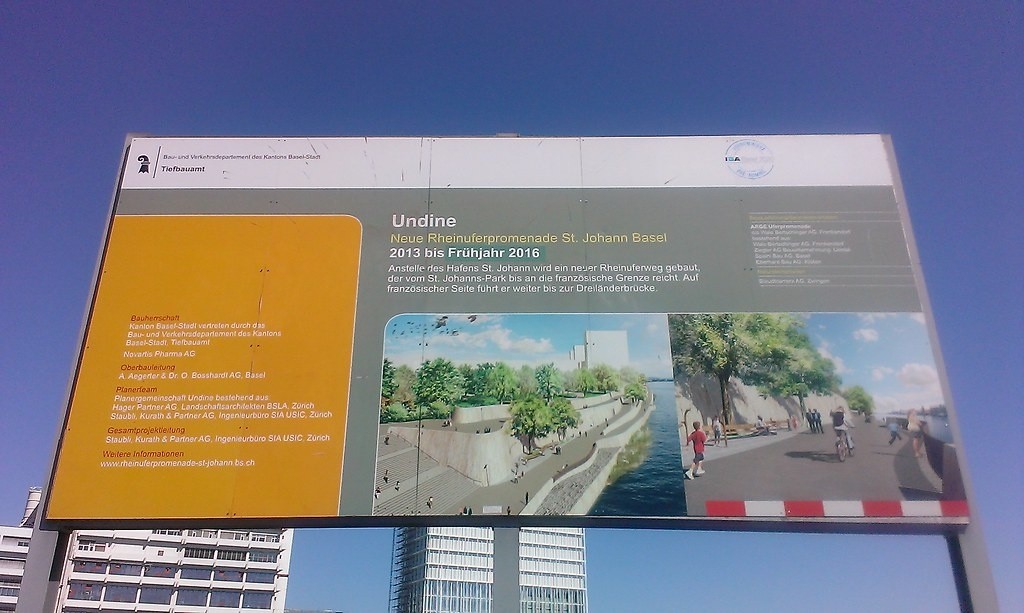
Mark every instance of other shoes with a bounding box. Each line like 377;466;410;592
696;470;705;476
685;470;695;480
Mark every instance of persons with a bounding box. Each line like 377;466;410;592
514;473;518;483
507;506;511;515
556;447;561;455
833;406;855;451
887;420;901;444
806;408;823;434
756;416;767;436
712;415;721;447
428;497;433;508
906;408;923;457
464;506;472;515
384;470;389;482
384;436;390;445
375;487;382;498
395;481;400;491
685;422;708;481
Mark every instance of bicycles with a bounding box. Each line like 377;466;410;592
835;428;855;463
